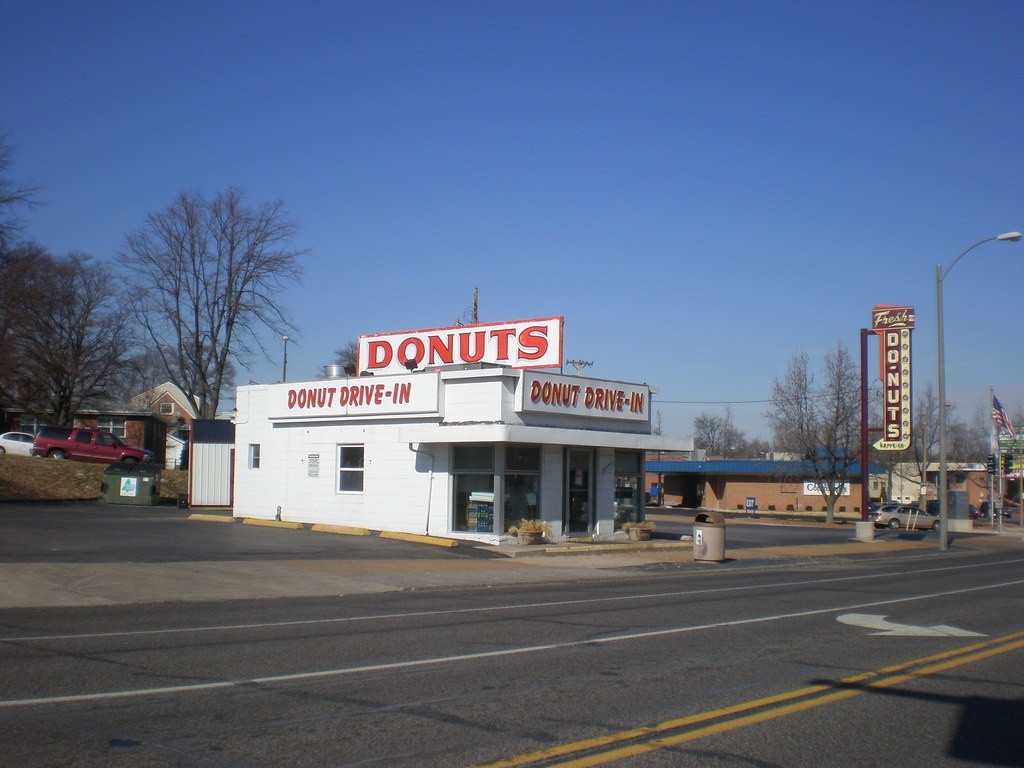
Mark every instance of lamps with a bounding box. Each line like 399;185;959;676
406;358;418;371
344;362;356;377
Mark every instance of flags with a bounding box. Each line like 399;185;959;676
992;394;1015;441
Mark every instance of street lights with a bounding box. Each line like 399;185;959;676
921;403;953;514
936;230;1023;551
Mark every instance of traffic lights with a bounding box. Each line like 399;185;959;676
1004;454;1013;475
987;454;996;475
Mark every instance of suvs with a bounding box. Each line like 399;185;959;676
868;506;941;532
32;425;154;464
980;502;1012;518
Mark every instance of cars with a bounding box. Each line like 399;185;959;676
969;505;979;519
0;432;40;458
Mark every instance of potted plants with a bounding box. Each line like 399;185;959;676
621;521;657;541
508;516;552;545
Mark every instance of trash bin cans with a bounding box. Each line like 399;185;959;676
692;512;726;563
102;461;164;505
